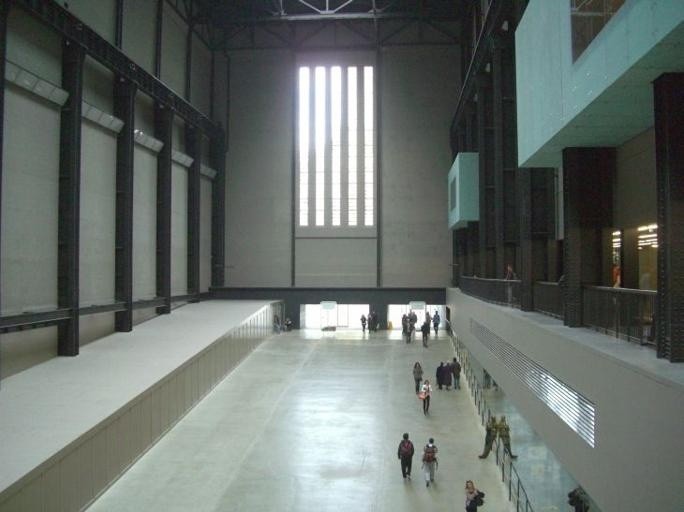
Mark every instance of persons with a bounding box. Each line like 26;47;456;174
498;414;517;459
360;309;497;512
639;265;655;320
271;314;293;334
505;263;520;305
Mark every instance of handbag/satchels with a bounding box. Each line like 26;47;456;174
418;393;427;400
470;491;484;510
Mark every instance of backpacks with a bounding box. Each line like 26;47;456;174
400;442;412;457
424;444;435;462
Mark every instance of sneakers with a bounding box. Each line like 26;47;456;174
478;455;486;458
511;456;517;458
